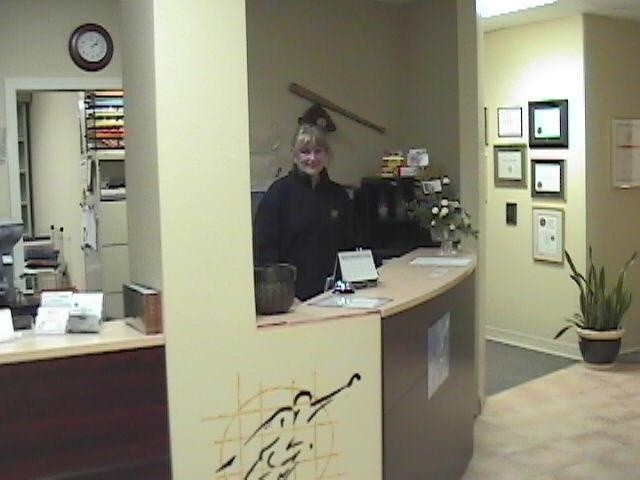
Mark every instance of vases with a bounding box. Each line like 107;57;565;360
429;226;465;258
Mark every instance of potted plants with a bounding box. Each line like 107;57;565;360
549;241;640;373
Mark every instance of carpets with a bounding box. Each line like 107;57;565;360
460;357;640;480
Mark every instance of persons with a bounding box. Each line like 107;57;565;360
253;124;350;301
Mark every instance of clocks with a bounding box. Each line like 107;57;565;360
67;22;115;73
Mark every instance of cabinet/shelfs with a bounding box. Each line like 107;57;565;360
31;90;131;322
16;100;32;236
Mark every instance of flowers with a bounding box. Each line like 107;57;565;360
403;190;482;249
